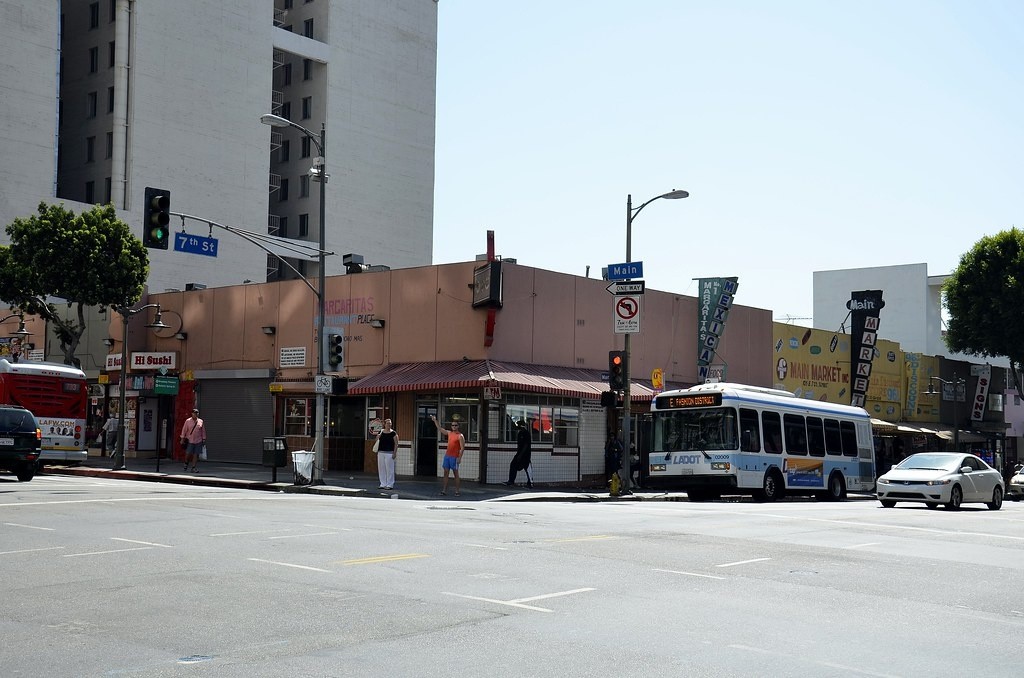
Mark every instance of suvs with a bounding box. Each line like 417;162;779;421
0;405;42;482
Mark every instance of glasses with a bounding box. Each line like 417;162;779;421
451;425;458;426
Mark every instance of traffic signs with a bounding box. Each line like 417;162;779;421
172;231;218;258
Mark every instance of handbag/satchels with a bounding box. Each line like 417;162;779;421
96;434;102;442
630;456;639;464
181;438;189;449
199;445;207;460
373;429;383;452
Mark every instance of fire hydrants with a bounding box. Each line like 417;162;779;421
609;473;621;495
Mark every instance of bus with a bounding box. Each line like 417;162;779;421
0;355;88;465
643;383;876;501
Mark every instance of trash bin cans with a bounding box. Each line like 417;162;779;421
291;452;316;485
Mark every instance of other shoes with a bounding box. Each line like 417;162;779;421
503;482;513;487
524;484;533;488
385;487;392;490
191;467;199;473
110;450;116;459
184;462;189;471
378;487;384;489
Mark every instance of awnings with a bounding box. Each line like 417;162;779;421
347;360;654;401
871;418;954;436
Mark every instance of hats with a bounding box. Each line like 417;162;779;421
191;409;199;413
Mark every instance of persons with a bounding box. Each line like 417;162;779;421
99;413;119;458
432;416;465;497
180;409;207;473
502;421;534;489
375;419;399;490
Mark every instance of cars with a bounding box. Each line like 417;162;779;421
876;452;1006;511
1009;465;1024;502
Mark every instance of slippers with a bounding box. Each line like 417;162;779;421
439;491;445;496
455;493;460;496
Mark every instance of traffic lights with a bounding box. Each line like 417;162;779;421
142;186;170;250
609;350;623;390
322;326;345;376
600;391;617;408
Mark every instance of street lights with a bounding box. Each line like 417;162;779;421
623;189;689;493
111;303;171;469
921;376;960;452
261;113;328;486
